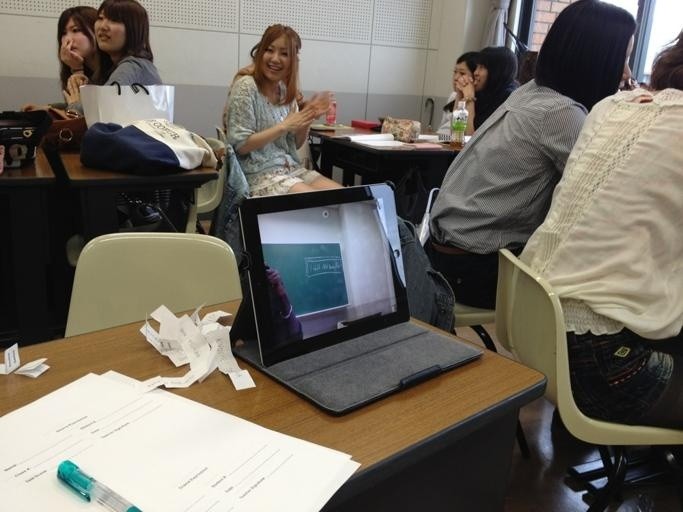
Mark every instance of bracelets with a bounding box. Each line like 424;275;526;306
461;96;474;105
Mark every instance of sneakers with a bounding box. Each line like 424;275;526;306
518;395;558;469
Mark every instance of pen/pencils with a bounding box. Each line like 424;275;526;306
57;459;143;512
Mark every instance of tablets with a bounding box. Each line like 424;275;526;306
237;183;411;368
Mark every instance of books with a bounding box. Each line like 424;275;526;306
333;132;406;150
309;123;355;136
419;133;472;145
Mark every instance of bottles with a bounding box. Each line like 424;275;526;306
325;93;337;126
451;102;469;148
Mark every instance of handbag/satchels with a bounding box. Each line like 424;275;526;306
79;118;224;176
79;84;175;128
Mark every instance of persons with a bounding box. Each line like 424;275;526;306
58;1;172;227
453;46;521;137
519;29;683;435
433;53;476;146
221;64;307;140
265;261;303;343
227;26;345;199
423;2;634;314
21;7;101;121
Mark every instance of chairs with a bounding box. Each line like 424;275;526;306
389;209;537;466
487;243;681;512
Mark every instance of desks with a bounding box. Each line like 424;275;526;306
0;292;554;510
